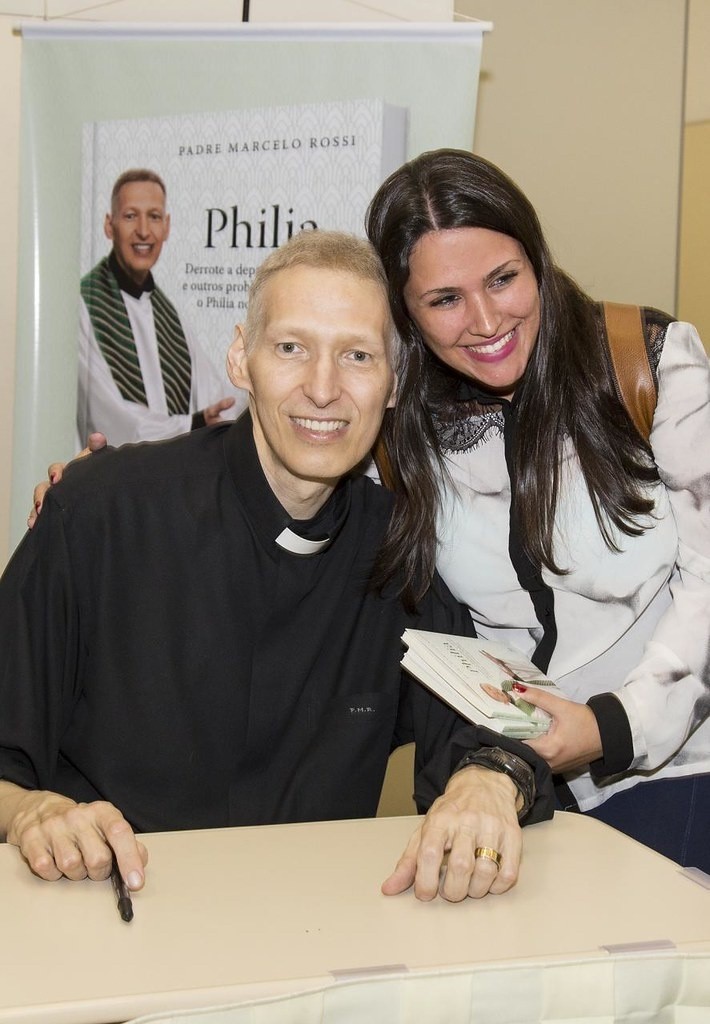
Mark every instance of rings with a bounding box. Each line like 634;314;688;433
475;848;501;871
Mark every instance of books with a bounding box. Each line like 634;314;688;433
399;628;564;742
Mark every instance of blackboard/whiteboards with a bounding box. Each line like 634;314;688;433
452;0;689;321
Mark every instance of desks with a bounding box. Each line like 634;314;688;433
0;806;710;1024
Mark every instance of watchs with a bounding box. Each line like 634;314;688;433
452;747;535;819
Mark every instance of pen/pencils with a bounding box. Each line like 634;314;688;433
110;851;134;922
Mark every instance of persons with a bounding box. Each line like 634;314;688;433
0;232;554;903
74;169;236;448
479;649;560;719
25;148;710;873
